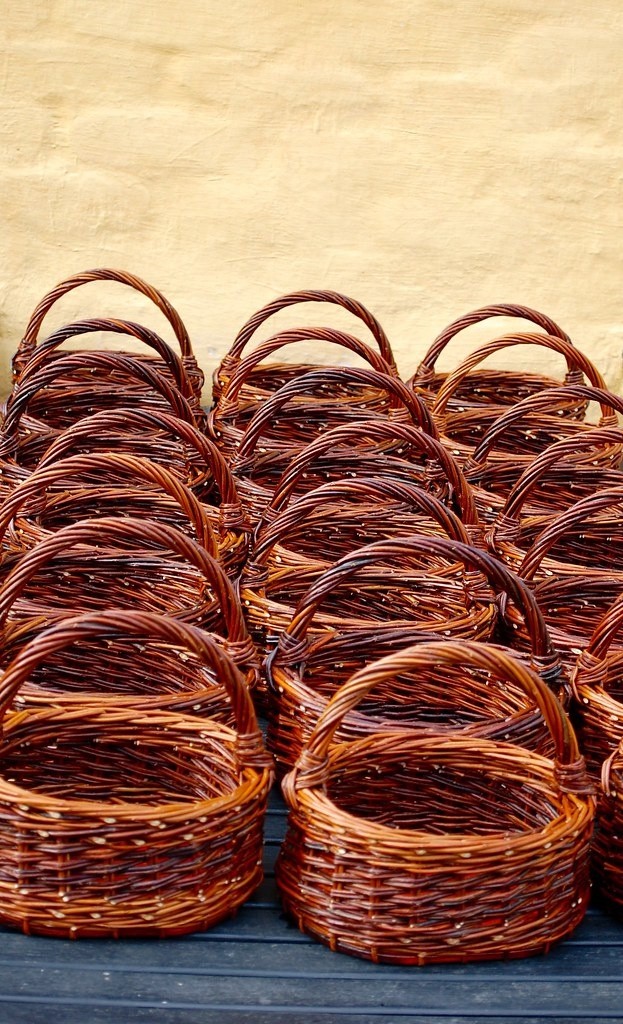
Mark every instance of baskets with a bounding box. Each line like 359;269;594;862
0;269;623;964
